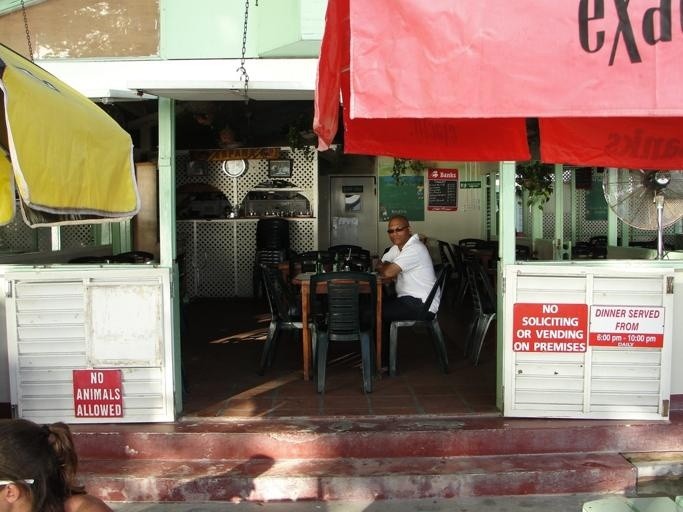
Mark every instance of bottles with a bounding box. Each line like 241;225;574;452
264;205;313;217
333;264;340;273
316;261;323;275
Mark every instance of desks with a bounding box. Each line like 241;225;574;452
294;269;392;382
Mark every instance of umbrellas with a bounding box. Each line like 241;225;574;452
0;43;142;230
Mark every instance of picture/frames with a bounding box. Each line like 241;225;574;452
268;159;292;178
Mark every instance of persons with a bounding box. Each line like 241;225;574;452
0;417;113;511
360;215;440;375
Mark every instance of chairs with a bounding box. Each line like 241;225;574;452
465;261;495;366
114;251;152;264
67;256;113;264
309;271;378;393
389;263;452;378
257;259;321;378
252;218;683;307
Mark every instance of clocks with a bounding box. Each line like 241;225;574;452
222;160;246;178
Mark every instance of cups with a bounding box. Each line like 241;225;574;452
344;266;350;271
355;264;363;272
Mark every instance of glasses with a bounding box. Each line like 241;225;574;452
387;226;407;233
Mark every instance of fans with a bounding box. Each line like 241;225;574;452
598;169;683;258
